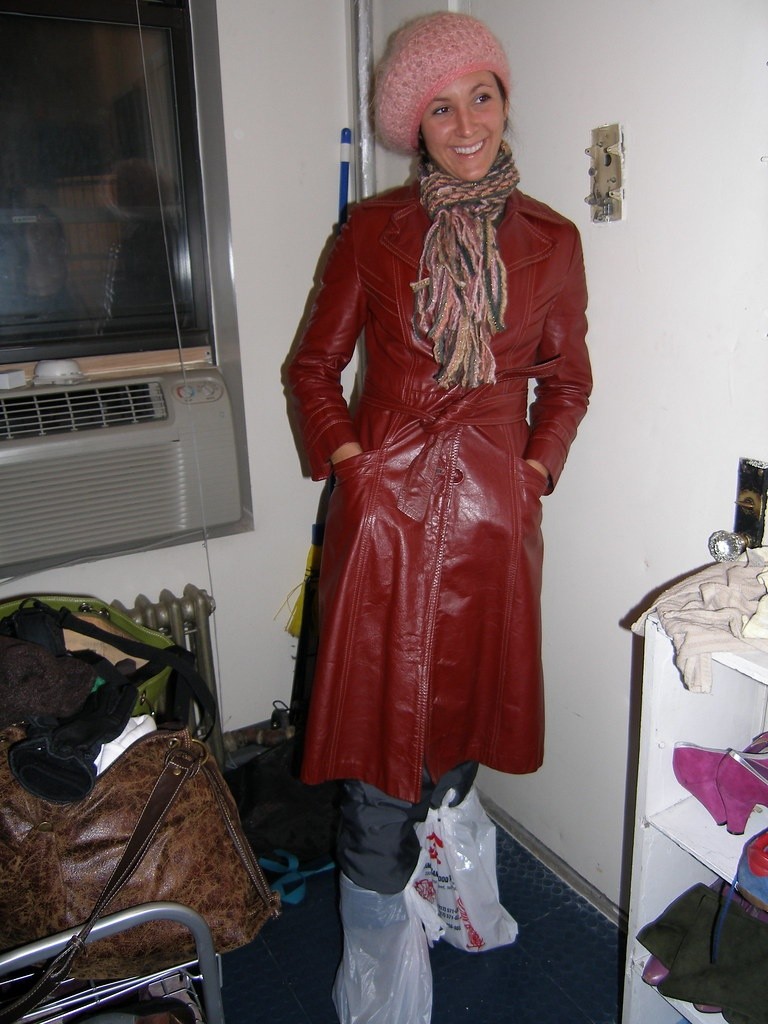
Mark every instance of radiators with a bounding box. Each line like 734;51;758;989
111;584;229;782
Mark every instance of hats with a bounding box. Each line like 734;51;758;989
370;11;514;159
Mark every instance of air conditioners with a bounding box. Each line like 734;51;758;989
0;367;243;569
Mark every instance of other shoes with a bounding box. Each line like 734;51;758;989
640;876;768;1015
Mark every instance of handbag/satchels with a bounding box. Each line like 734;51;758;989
0;718;291;982
1;594;191;722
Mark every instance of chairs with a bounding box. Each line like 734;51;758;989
0;612;228;1024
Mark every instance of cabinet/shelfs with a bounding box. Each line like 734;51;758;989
623;599;768;1024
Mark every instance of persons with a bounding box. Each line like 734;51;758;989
285;11;594;1024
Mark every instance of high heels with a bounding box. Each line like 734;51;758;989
673;733;768;836
